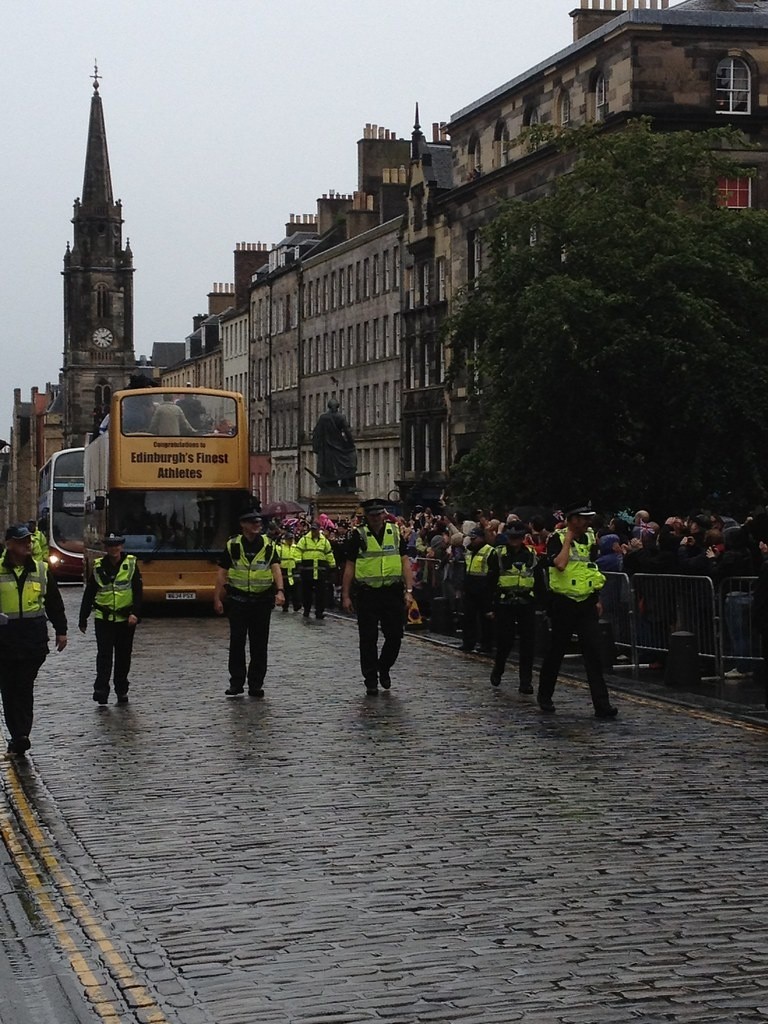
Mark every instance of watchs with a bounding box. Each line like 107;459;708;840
277;589;285;595
406;589;412;593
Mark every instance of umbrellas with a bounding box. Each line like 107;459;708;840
259;501;305;516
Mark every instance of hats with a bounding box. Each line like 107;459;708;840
285;533;293;539
362;499;389;515
236;506;262;522
562;501;596;516
505;522;527;534
690;514;710;529
5;524;36;541
102;529;126;545
309;523;320;529
468;527;485;538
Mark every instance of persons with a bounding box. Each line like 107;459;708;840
1;518;50;562
99;394;238;436
0;524;68;754
312;398;358;488
78;529;142;705
342;499;413;695
537;500;618;718
268;509;768;678
482;520;547;694
214;508;286;696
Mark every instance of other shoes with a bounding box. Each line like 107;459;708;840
282;604;324;619
724;668;742;677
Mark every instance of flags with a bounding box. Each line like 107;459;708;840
640;518;655;536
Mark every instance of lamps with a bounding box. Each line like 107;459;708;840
258;410;264;416
330;375;339;388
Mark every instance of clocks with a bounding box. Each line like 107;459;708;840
91;327;115;350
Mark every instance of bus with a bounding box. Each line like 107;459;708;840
36;446;84;586
83;387;251;611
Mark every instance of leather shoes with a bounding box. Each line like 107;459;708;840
595;707;618;717
12;734;31;753
491;666;505;686
93;690;107;704
537;693;556;713
377;660;391;689
519;683;533;694
226;686;243;695
457;645;475;651
367;687;379;696
249;689;264;697
118;694;128;702
